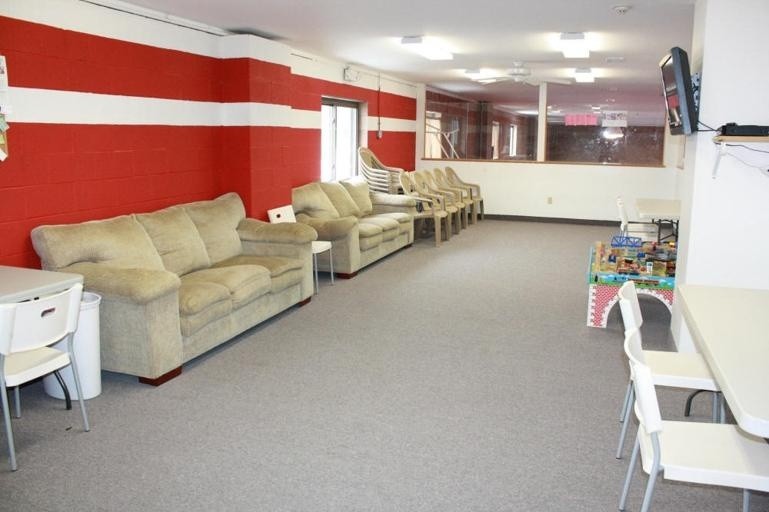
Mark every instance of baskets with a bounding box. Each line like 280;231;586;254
611;236;642;247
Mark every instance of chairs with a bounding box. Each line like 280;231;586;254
263;205;336;294
355;143;485;248
1;279;92;471
615;198;663;246
615;277;769;512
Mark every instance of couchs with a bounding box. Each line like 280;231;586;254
29;188;318;385
289;175;419;282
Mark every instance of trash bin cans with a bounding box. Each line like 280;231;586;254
41;292;103;400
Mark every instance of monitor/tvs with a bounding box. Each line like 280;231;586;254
658;46;696;135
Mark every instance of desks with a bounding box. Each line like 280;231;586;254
1;263;85;420
635;193;680;241
